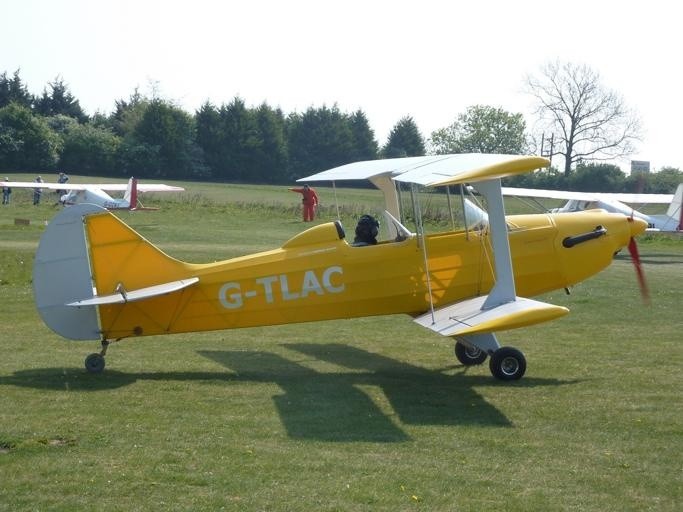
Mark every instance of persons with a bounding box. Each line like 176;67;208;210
351;214;380;247
1;177;11;205
31;176;43;206
55;172;68;205
287;183;318;223
